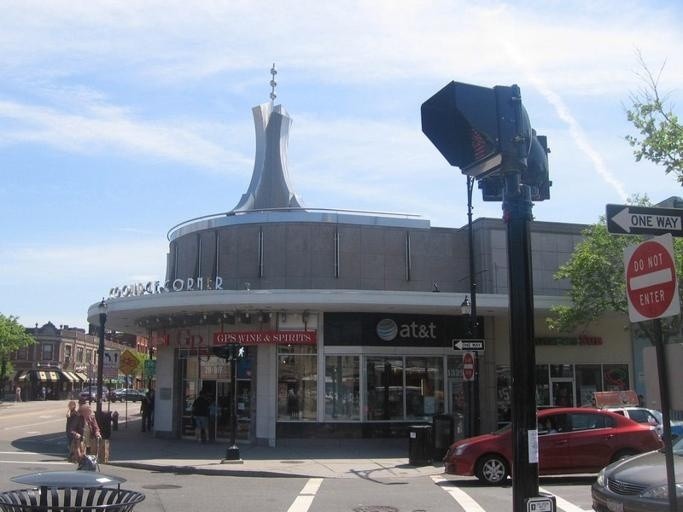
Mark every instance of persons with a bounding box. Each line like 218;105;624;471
65;401;81;459
139;392;150;431
14;385;21;401
538;417;558;434
191;388;211;442
68;404;102;468
77;399;86;418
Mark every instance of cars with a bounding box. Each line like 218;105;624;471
600;406;673;445
588;430;683;512
78;385;147;402
441;406;665;486
661;421;683;442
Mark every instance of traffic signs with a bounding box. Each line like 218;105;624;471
452;338;486;352
606;203;683;236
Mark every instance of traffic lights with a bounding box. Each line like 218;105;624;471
418;79;504;175
232;344;248;359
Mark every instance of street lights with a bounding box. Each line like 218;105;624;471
457;296;476;437
94;299;107;435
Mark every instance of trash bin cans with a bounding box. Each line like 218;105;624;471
409;411;464;466
0;469;146;512
95;410;111;438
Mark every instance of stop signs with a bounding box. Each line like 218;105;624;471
617;232;680;326
461;350;475;381
